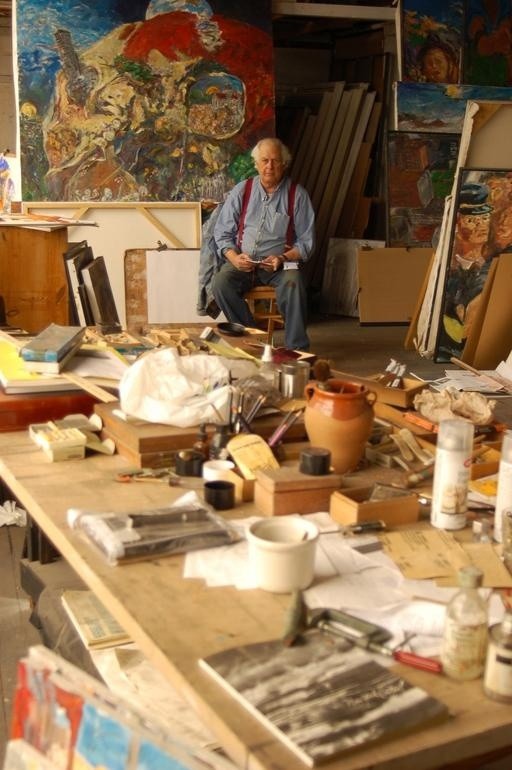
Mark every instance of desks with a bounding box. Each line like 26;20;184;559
0;323;512;770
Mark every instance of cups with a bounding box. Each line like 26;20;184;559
246;518;319;593
282;360;310;400
299;448;330;475
175;449;234;510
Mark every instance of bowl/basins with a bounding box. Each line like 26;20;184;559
217;322;246;337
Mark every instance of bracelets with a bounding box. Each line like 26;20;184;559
282;254;289;262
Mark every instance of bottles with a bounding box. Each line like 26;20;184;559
258;344;280;390
193;422;230;460
441;566;512;702
304;380;377;476
431;420;512;543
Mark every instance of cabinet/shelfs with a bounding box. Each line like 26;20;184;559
0;228;71;330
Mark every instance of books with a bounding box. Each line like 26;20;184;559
0;336;134;396
196;631;448;769
65;246;121;328
61;589;136;651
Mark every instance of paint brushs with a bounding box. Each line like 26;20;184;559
402;422;506;488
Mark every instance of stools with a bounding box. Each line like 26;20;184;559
242;287;285;341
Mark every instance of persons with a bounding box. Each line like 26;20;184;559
210;137;317;353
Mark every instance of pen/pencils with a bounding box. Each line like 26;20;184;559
133;475;188;485
212;388;303;450
